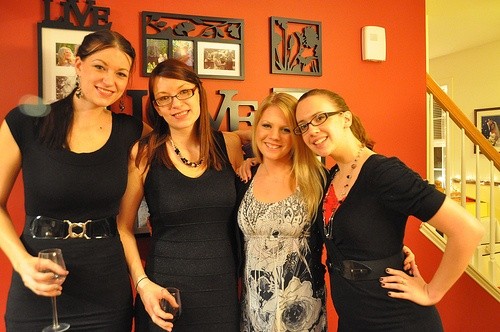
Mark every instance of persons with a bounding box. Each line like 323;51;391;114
117;58;247;332
207;51;233;70
0;29;252;332
483;118;499;146
235;89;486;332
58;44;73;65
147;92;415;332
175;45;192;66
158;53;167;62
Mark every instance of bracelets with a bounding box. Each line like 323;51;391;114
135;276;148;292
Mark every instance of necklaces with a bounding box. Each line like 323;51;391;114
167;136;203;167
322;144;365;240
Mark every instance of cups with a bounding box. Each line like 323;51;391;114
159;287;182;332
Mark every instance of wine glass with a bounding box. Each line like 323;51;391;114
38;248;70;332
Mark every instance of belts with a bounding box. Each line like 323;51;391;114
326;253;405;280
22;213;121;240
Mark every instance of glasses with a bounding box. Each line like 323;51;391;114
154;84;198;106
293;111;346;135
